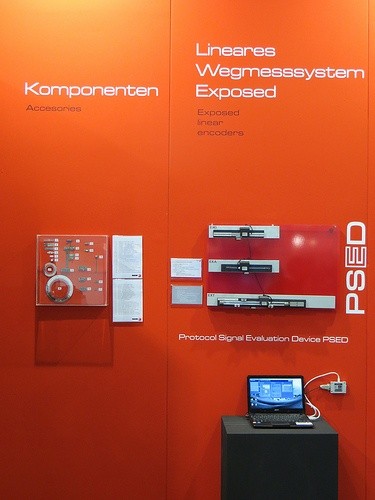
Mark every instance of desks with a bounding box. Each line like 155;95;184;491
219;414;339;500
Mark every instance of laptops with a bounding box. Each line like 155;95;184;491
247;374;314;429
